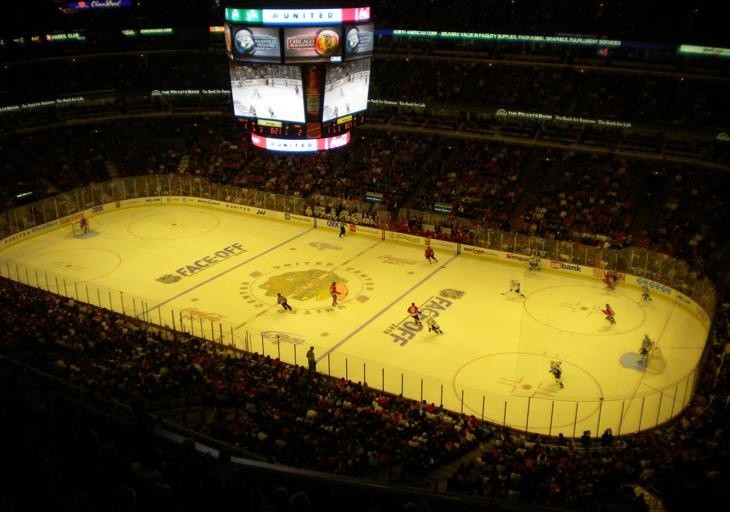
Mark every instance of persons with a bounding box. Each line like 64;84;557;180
329;281;341;307
227;29;730;243
483;243;729;339
427;315;444;335
276;292;292;311
1;36;225;511
407;302;422;327
424;246;439;265
225;339;730;511
